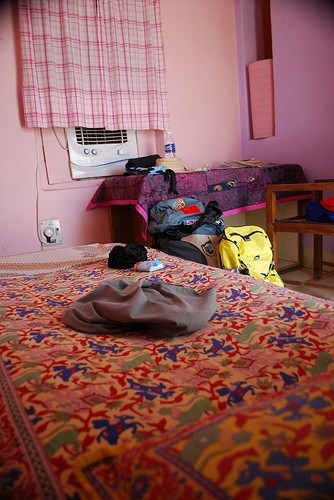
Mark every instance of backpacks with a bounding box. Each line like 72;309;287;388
218;224;284;288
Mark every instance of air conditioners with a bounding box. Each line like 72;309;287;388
63;127;139;179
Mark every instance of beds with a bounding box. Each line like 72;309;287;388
0;243;334;500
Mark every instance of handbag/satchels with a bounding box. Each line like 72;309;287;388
148;197;204;235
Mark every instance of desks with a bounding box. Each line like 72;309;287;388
86;164;311;275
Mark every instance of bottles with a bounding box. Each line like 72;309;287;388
164;131;175;158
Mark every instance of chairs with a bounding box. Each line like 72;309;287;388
266;179;334;290
